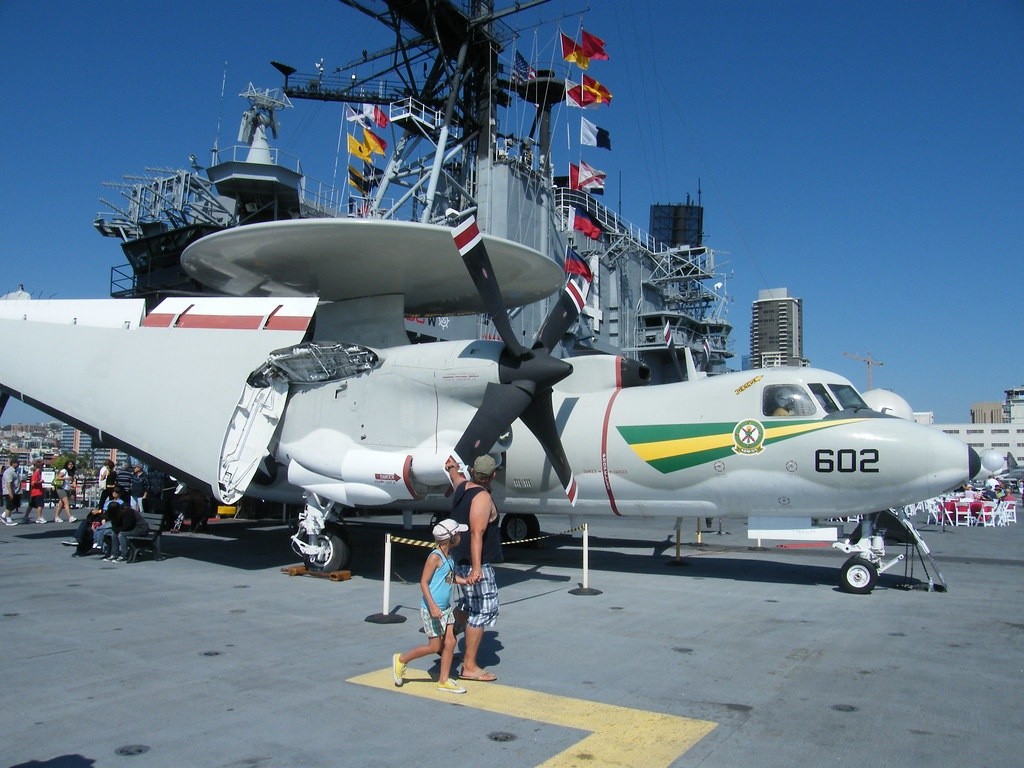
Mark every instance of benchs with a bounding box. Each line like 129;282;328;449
86;512;165;563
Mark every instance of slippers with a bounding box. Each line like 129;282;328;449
459;671;498;681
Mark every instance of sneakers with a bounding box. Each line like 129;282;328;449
69;516;78;523
54;517;63;523
36;517;47;524
436;678;468;694
103;555;118;561
112;556;128;564
23;517;33;523
392;653;408;686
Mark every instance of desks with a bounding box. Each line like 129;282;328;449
938;502;992;525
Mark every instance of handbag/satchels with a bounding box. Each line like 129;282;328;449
51;469;65;487
98;470;107;490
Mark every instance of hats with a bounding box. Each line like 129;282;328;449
132;463;143;470
994;486;1002;491
433;518;468;540
473;454;496;475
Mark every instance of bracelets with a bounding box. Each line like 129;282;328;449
448;465;454;472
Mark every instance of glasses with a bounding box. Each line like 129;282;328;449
113;490;118;493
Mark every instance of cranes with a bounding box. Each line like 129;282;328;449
841;348;884;390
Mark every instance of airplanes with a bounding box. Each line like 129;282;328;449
0;214;984;593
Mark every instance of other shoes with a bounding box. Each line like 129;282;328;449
0;515;7;525
92;542;98;548
6;521;18;526
97;545;102;549
61;540;80;546
72;551;85;557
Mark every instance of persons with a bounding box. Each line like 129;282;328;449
772;388;795;416
965;475;1015;509
0;460;23;526
98;459;149;513
54;460;77;523
393;519;480;694
72;485;149;563
437;455;500;681
23;461;47;523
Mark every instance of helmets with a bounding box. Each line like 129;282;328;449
775;388;795;410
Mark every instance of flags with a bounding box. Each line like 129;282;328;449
512;50;535;84
582;74;612;108
346;103;390;192
581;117;611;151
570;160;606;196
581;29;609;60
565;78;600;109
564;246;591;284
568;205;604;239
561;32;590;70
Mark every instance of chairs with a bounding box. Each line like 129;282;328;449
892;491;1017;528
